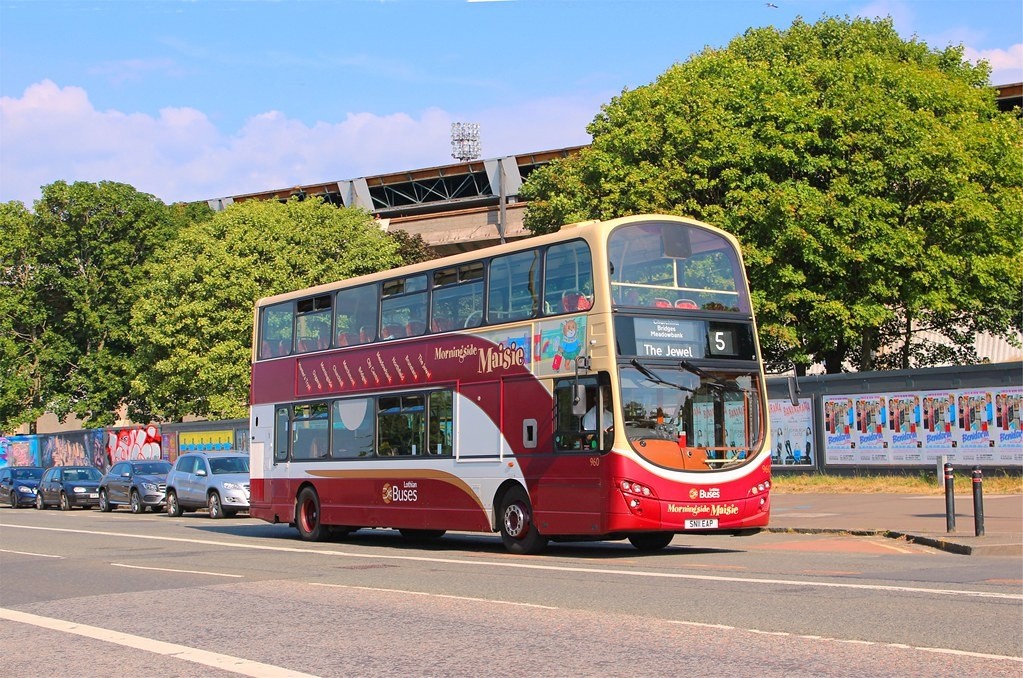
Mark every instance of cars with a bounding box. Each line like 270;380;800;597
99;460;173;514
0;466;47;509
37;466;105;511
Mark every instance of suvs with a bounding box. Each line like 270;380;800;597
165;450;250;519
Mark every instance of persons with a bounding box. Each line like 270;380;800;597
698;427;812;465
378;415;445;457
582;386;613;443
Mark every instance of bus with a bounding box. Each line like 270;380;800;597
251;210;801;555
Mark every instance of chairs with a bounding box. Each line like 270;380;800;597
261;288;696;359
